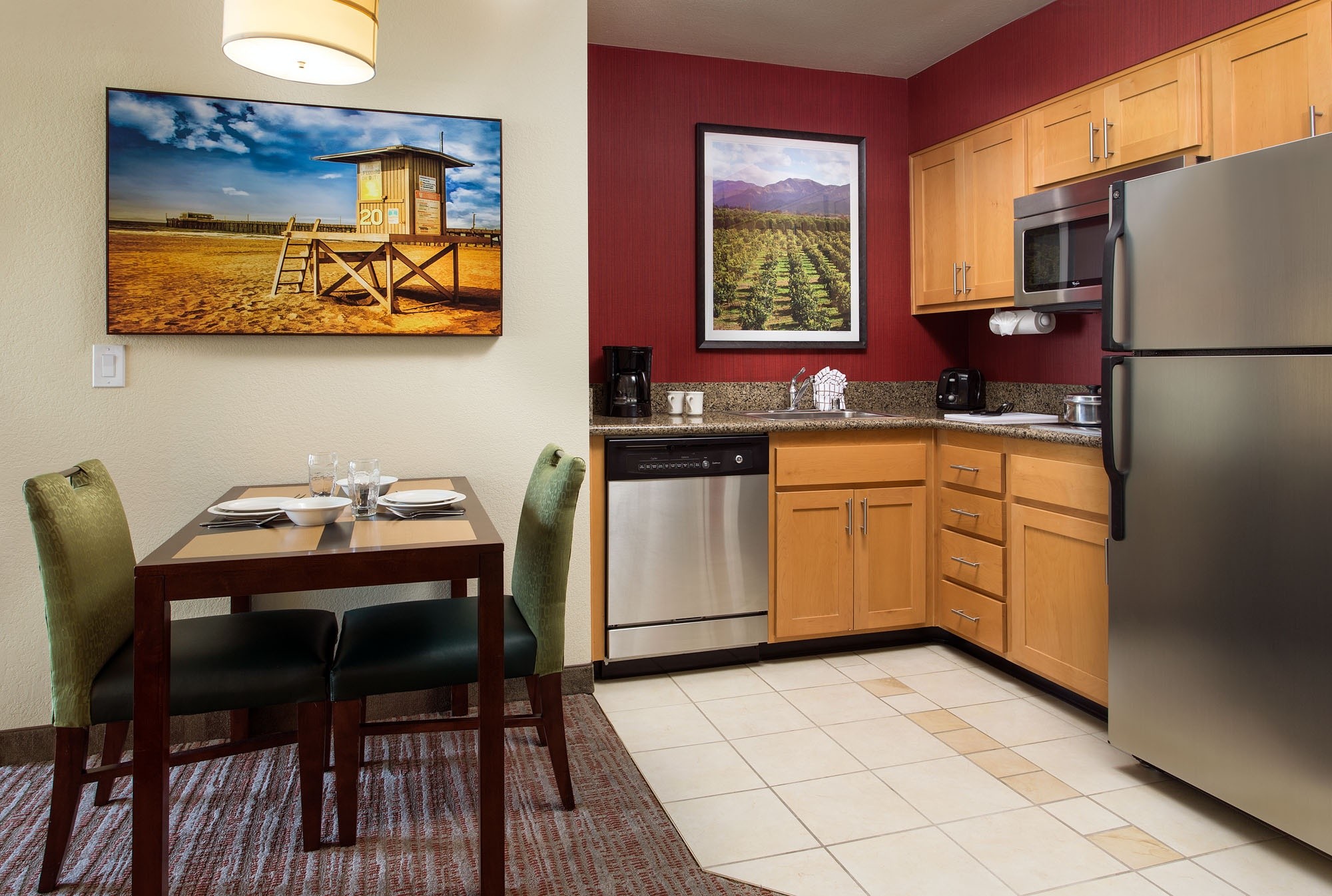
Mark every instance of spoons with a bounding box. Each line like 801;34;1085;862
207;513;281;528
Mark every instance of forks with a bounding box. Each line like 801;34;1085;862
385;507;464;518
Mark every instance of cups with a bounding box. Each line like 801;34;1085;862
348;458;378;518
308;452;339;497
666;391;684;415
685;391;704;416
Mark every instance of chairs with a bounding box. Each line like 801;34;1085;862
22;457;338;894
329;442;587;848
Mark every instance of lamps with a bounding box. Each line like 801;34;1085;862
223;0;379;86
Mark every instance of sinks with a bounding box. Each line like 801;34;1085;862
722;410;900;422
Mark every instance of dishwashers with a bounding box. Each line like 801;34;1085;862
604;435;770;664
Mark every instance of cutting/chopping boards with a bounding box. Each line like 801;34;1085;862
943;411;1059;422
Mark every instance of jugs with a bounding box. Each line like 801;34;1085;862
612;370;650;405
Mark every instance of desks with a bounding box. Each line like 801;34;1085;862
132;475;506;896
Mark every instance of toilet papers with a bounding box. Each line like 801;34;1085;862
989;308;1057;337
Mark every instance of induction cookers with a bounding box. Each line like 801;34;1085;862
1031;423;1101;437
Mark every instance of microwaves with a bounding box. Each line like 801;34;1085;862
1014;156;1186;313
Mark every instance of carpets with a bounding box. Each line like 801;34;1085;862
0;692;786;896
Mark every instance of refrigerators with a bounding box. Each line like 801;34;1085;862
1102;132;1331;862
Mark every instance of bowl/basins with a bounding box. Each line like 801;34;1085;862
277;497;353;526
335;476;398;498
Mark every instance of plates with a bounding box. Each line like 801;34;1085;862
207;497;297;519
376;490;466;511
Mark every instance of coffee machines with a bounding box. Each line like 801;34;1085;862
603;345;652;417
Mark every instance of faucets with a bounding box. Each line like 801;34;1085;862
787;367;816;410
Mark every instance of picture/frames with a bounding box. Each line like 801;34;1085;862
695;120;868;353
104;86;504;337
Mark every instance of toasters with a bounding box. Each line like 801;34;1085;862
936;367;986;410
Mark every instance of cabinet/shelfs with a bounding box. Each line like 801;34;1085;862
909;123;1027;308
768;431;932;644
936;442;1007;654
1006;451;1110;706
1024;50;1204;193
1211;0;1332;163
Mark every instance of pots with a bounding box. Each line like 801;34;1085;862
1064;385;1102;424
